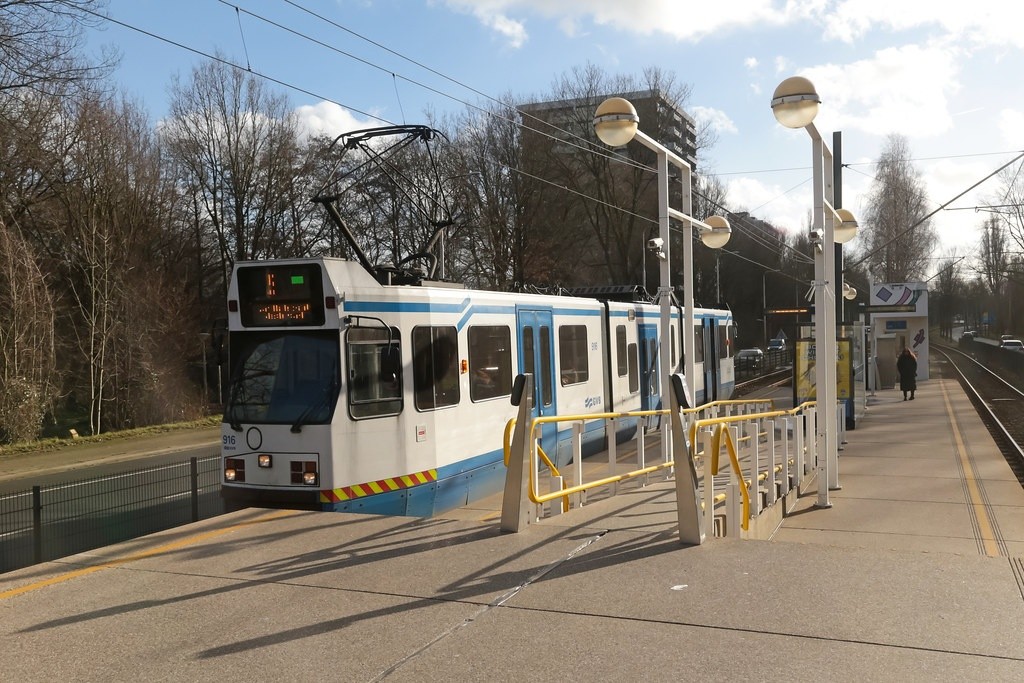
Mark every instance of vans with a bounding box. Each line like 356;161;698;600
765;338;787;354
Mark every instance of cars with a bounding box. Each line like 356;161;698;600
1000;335;1014;346
962;331;973;341
1001;339;1024;354
734;348;765;371
970;331;978;338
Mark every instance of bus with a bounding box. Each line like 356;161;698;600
217;124;737;520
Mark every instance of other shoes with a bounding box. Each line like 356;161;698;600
909;397;914;400
904;397;907;401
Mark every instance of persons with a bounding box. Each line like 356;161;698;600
897;347;917;401
